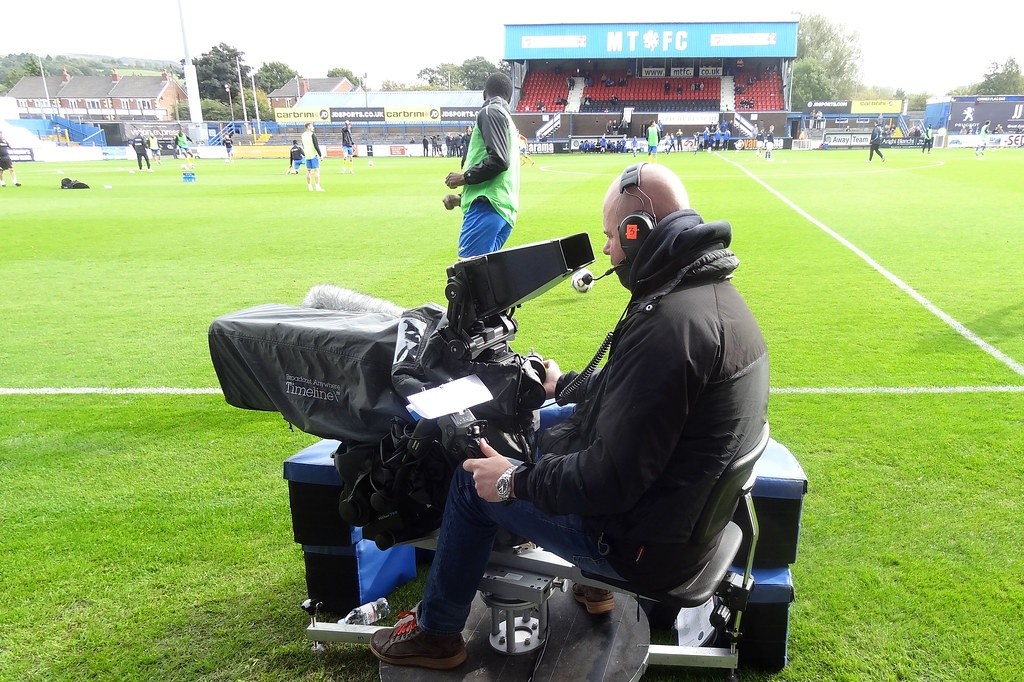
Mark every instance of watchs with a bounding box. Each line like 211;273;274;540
495;466;519;502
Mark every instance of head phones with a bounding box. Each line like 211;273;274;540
618;161;658;266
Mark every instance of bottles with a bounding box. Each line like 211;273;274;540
338;597;390;627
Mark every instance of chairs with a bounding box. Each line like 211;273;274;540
515;67;784;113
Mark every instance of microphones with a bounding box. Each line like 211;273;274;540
301;284;405;320
582;258;631;285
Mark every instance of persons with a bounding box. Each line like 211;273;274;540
132;132;154;172
866;120;885;162
808;109;823;129
175;130;193;158
0;131;21;187
579;119;733;158
409;138;415;144
370;162;770;669
301;123;325;191
289;140;305;174
537;61;779;113
443;73;519;259
914;124;931;153
975;120;991;156
148;133;160;161
422;126;473;170
341;120;355;175
754;123;775;159
222;135;233;158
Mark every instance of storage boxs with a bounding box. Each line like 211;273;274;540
283;438;417;610
721;436;807;670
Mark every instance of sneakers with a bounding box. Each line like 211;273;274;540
571;582;615;614
368;600;467;668
